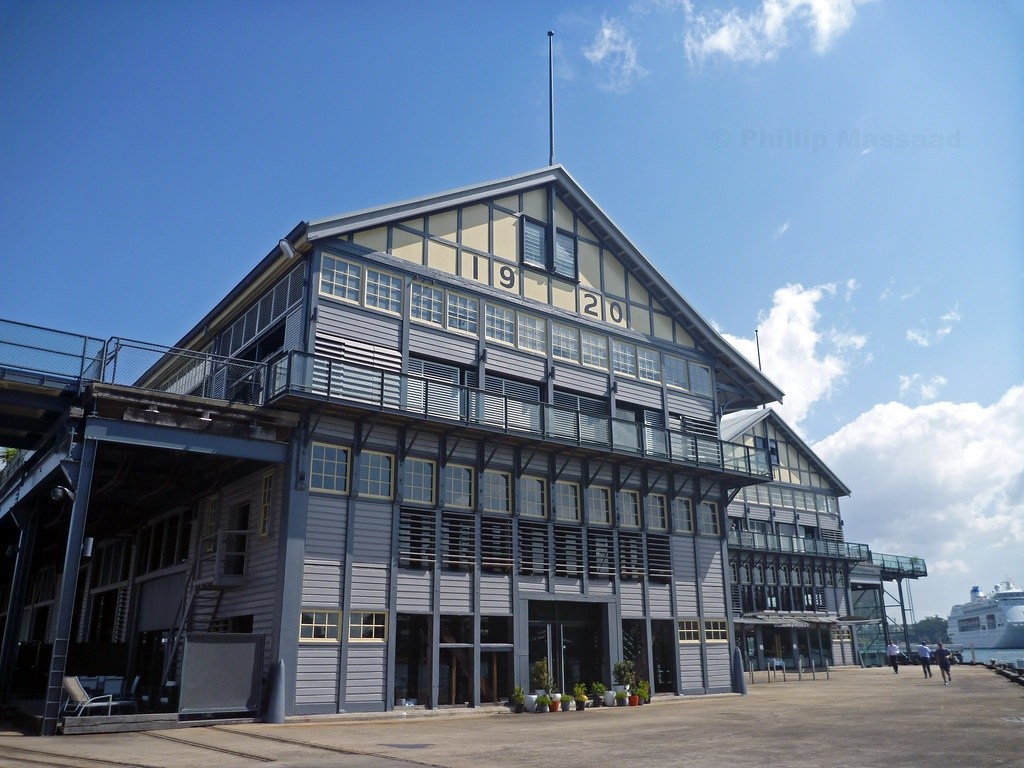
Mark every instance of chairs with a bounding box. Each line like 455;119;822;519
62;676;140;717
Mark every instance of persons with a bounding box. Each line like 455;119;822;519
918;641;932;679
736;636;742;647
800;644;809;657
934;642;951;686
886;640;900;674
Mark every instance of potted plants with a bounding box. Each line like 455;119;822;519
510;657;653;714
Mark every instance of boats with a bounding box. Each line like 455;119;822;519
947;581;1024;649
897;642;964;660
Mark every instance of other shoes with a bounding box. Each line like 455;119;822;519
949;676;951;681
944;682;948;686
895;671;898;674
929;673;931;677
925;675;927;678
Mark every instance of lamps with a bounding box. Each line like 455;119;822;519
549;367;555;379
312;307;319;322
840;520;844;525
747;508;750;513
716;407;722;417
279;239;311;262
5;546;21;556
772;511;775;517
796;514;799;519
481;349;487;363
81;538;94;557
51;486;76;501
612;382;617;393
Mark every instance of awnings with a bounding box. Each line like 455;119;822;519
797;617;838;623
732;617;787;624
834;615;881;624
758;615;809;628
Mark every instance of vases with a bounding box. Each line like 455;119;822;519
604;691;616;706
524;695;538;711
